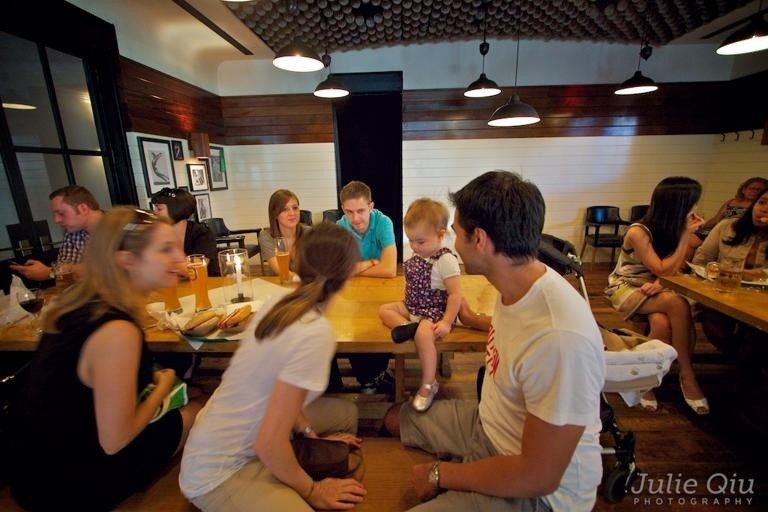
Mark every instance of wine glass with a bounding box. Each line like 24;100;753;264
16;288;45;336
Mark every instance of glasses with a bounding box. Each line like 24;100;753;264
119;209;151;250
161;187;177;200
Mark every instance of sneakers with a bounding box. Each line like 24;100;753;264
360;368;395;395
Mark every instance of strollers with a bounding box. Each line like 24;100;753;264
534;225;681;505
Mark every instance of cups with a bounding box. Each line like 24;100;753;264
164;288;183;314
51;259;75;291
713;256;746;292
704;262;719;279
185;254;212;313
275;236;292;285
217;249;253;304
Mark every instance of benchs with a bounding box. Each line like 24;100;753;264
590;308;736;365
0;436;460;512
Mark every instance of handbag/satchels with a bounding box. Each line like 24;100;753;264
291;438;349;481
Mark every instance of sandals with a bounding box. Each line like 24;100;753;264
640;398;657;411
680;375;710;415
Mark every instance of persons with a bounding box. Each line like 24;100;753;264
178;220;367;512
6;206;202;512
378;198;461;412
605;177;768;415
384;172;605;512
9;180;397;395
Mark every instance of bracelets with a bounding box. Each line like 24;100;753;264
303;424;314;438
469;313;485;328
304;481;314;500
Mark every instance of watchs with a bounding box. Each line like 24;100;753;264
427;461;439;490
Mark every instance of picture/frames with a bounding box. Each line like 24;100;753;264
207;146;228;191
193;194;213;223
172;141;184;161
137;135;177;197
186;163;209;191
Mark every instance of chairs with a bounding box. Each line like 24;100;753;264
323;209;341;223
580;206;631;271
632;205;649;223
201;218;262;272
300;210;312;226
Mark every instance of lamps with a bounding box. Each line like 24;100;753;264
273;0;325;73
463;9;502;98
714;0;768;56
314;53;351;98
614;32;659;96
188;132;210;159
487;11;540;126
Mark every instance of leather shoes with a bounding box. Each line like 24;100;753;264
392;322;418;343
413;378;439;412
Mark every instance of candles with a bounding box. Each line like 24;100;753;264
234;257;244;294
